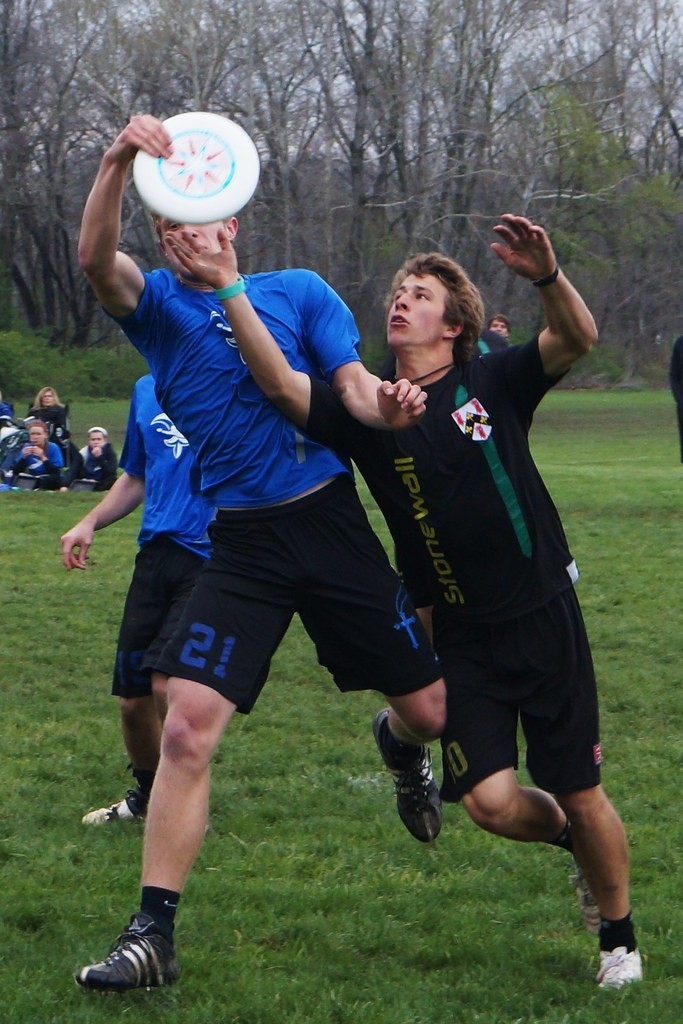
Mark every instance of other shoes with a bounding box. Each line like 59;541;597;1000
82;787;147;826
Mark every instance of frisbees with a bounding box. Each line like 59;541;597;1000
132;111;262;225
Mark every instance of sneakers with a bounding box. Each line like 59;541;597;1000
576;872;601;934
76;913;181;994
371;706;442;843
596;947;644;992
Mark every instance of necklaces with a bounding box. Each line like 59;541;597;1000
394;362;455;382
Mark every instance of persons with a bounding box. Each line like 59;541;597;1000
71;112;446;993
165;214;644;990
669;336;683;464
487;315;511;340
0;386;117;493
60;375;217;828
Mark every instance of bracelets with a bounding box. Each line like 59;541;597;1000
213;278;245;300
529;262;559;288
